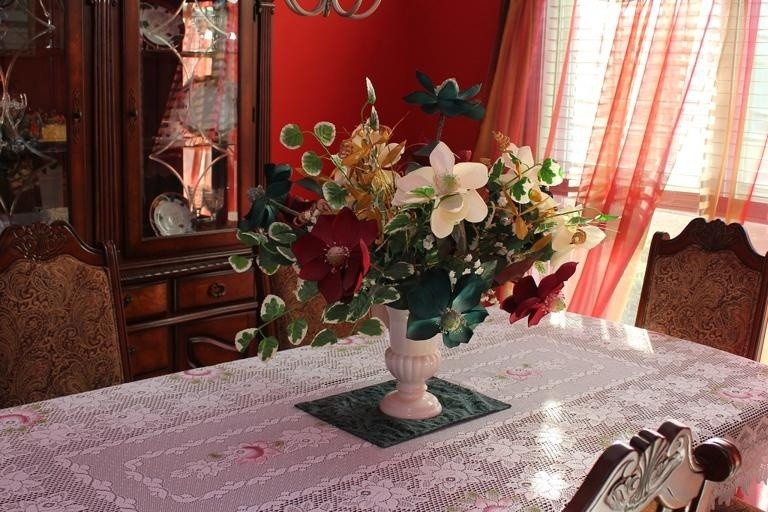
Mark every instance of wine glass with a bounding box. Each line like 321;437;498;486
186;188;206;219
205;189;225;222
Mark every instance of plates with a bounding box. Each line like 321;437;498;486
149;192;198;236
139;5;186;49
177;75;230;139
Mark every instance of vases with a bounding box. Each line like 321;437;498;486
380;307;442;420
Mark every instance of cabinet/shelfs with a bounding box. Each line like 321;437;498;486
0;1;275;381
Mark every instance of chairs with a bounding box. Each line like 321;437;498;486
635;217;768;363
0;219;131;408
562;419;741;512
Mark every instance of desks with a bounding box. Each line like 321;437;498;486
0;304;768;511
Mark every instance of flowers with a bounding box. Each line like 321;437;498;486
229;71;620;363
